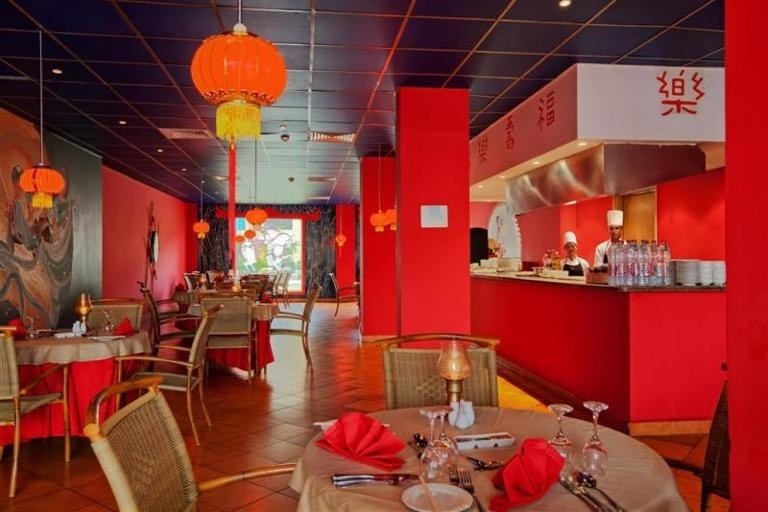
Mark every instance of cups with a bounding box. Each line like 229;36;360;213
470;260;496;270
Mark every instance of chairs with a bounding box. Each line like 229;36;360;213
137;269;322;386
664;381;729;512
83;375;297;511
329;273;360;317
86;297;144;330
114;304;225;446
0;326;72;498
374;333;501;409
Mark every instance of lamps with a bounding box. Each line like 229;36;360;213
435;341;474;418
75;292;92;333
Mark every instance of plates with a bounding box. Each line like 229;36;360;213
400;481;473;512
675;259;728;287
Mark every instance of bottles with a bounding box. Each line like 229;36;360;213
543;248;563;269
607;239;673;287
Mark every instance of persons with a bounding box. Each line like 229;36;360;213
561;231;591;275
592;209;627;267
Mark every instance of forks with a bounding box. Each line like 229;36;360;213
447;464;489;512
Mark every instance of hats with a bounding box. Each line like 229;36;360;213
564;231;577;246
608;211;623;226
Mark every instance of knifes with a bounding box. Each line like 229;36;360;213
330;472;421;480
557;472;620;508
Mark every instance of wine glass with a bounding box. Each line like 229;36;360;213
418;407;457;480
26;314;39;343
548;404;574;466
581;401;609;476
100;309;114;342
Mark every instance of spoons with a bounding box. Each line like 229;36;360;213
575;469;629;512
